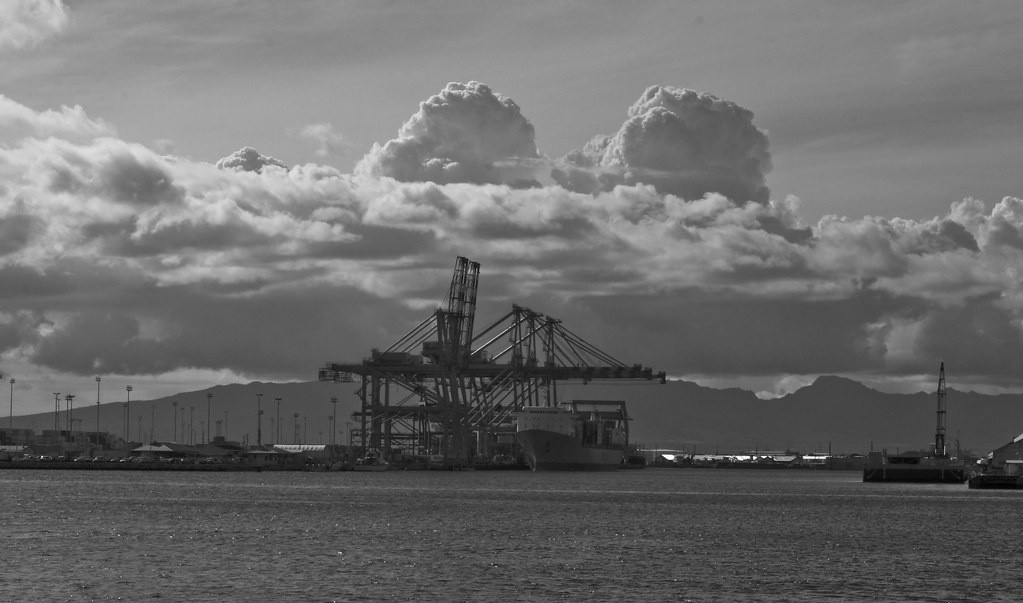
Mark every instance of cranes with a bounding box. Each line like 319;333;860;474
319;255;668;460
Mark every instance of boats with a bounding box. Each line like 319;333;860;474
862;362;969;485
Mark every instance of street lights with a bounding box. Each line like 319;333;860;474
304;415;307;444
125;383;133;442
173;402;178;443
256;392;264;451
9;376;16;429
180;407;184;441
189;406;194;444
206;393;214;444
225;410;228;441
95;375;102;451
330;398;338;443
152;405;156;441
276;397;282;444
294;412;298;445
